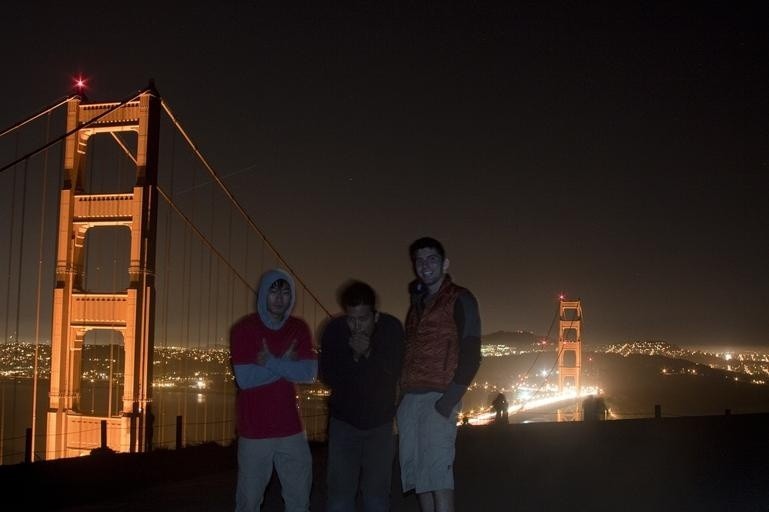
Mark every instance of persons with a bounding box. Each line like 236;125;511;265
492;394;508;421
392;237;481;512
230;268;321;512
318;281;406;511
582;395;608;423
